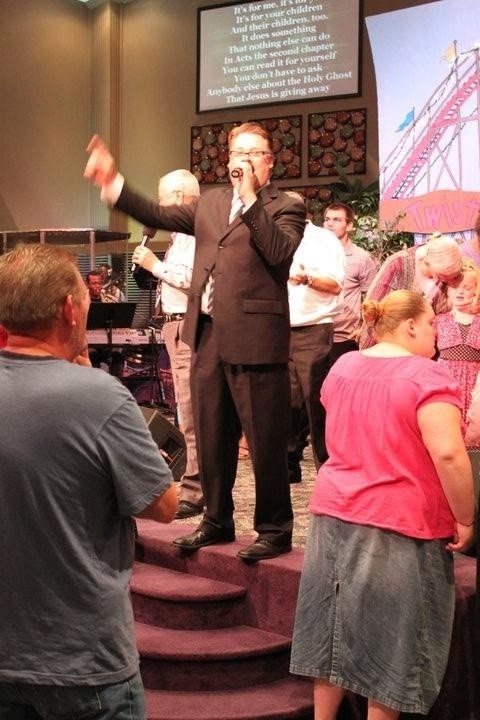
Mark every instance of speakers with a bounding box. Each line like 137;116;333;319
139;406;187;482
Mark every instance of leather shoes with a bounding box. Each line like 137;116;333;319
172;529;235;549
236;538;292;559
174;500;203;519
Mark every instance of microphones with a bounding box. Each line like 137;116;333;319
130;226;157;273
231;164;254;178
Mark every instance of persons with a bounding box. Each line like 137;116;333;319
288;289;479;720
82;117;308;561
1;169;479;520
0;240;180;717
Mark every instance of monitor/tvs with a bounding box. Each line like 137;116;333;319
196;0;362;115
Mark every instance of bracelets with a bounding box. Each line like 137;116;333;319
455;518;476;528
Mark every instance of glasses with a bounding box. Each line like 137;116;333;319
228;150;266;158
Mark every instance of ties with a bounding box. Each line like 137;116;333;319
208;203;246;318
155;231;177;308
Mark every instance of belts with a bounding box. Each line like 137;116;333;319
164;313;184;323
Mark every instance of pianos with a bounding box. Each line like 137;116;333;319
84;328;165;348
